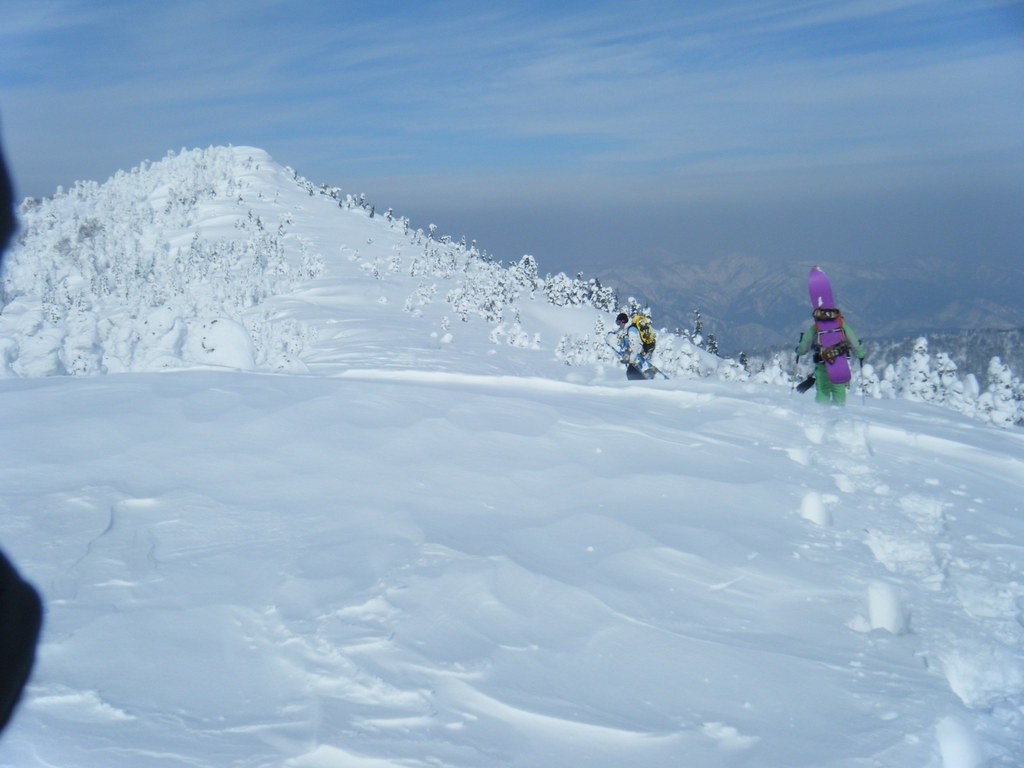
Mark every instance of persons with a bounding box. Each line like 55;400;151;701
796;315;866;408
616;313;647;380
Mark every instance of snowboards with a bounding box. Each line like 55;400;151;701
605;331;669;380
808;264;852;384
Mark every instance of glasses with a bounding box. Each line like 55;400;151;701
616;320;626;325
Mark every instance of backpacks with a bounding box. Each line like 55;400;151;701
628;313;655;353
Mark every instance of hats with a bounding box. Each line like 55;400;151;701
617;313;628;323
